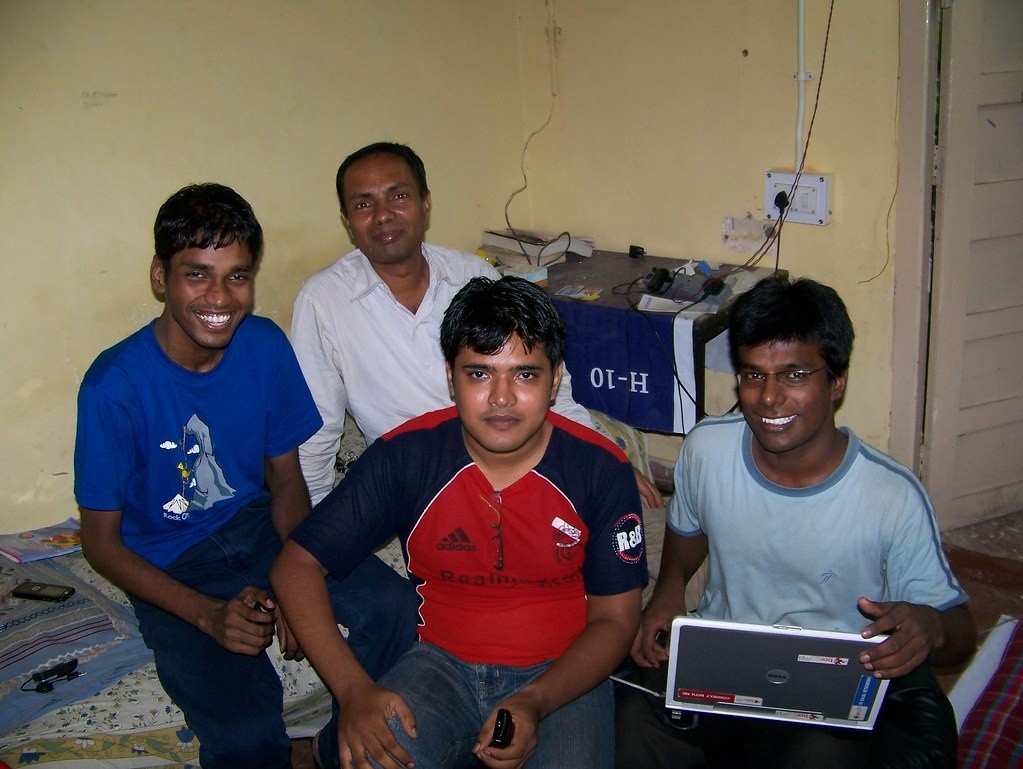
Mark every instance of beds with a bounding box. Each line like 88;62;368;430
1;413;714;769
945;615;1023;769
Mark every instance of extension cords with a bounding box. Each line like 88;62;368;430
642;271;734;306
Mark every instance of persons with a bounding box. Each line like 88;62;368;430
291;142;669;577
73;182;419;769
630;276;977;769
268;270;650;769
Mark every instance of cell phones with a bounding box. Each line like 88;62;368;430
491;709;513;749
10;582;76;603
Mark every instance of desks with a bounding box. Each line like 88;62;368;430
475;244;792;436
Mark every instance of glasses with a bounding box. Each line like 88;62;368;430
480;492;504;569
734;365;827;390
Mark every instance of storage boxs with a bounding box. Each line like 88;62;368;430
504;263;549;289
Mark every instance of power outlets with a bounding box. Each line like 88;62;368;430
764;170;835;226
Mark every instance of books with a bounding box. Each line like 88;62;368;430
480;228;594;268
0;517;82;564
